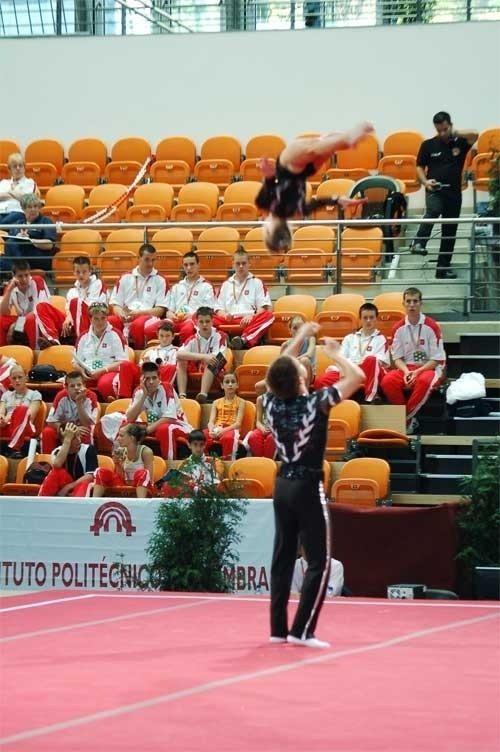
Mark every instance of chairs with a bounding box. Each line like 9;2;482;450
0;128;500;281
1;292;412;505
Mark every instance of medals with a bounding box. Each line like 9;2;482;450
415;346;421;352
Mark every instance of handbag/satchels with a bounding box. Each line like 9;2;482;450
452;399;492;417
28;364;59;380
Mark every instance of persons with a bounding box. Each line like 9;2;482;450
254;315;315;396
161;430;225;496
0;244;274;402
255;119;377;251
93;423;157;497
410;112;476;277
5;194;57;270
379;287;447;434
115;362;275;462
314;303;387;403
267;322;366;650
0;366;101;497
1;154;39;230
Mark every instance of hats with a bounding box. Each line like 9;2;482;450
89;302;109;315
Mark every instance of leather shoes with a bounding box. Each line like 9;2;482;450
410;243;427;256
436;272;457;278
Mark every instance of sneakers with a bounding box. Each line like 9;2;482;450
36;337;59;349
407;417;420;435
195;392;208;404
232;336;243;349
286;635;330;649
